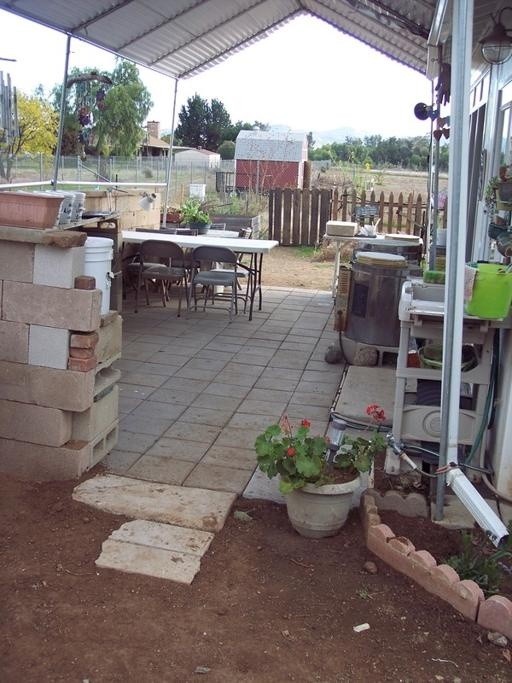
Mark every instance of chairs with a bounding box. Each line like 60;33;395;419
122;226;255;324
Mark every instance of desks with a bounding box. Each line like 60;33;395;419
121;230;280;321
322;231;424;297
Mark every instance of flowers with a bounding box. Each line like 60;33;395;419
253;402;393;496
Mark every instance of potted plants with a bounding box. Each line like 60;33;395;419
160;195;269;240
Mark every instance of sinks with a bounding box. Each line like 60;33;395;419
410;283;445;303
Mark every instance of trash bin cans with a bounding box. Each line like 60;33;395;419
78;212;123;314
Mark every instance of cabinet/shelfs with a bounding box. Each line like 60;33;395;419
61;210;124;316
382;279;512;484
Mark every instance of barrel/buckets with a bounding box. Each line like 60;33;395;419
465;263;511;320
85;235;114;316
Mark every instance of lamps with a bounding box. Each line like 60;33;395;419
478;5;512;67
137;192;157;213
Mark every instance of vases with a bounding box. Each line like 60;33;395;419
279;469;363;540
0;190;88;230
487;179;511;259
